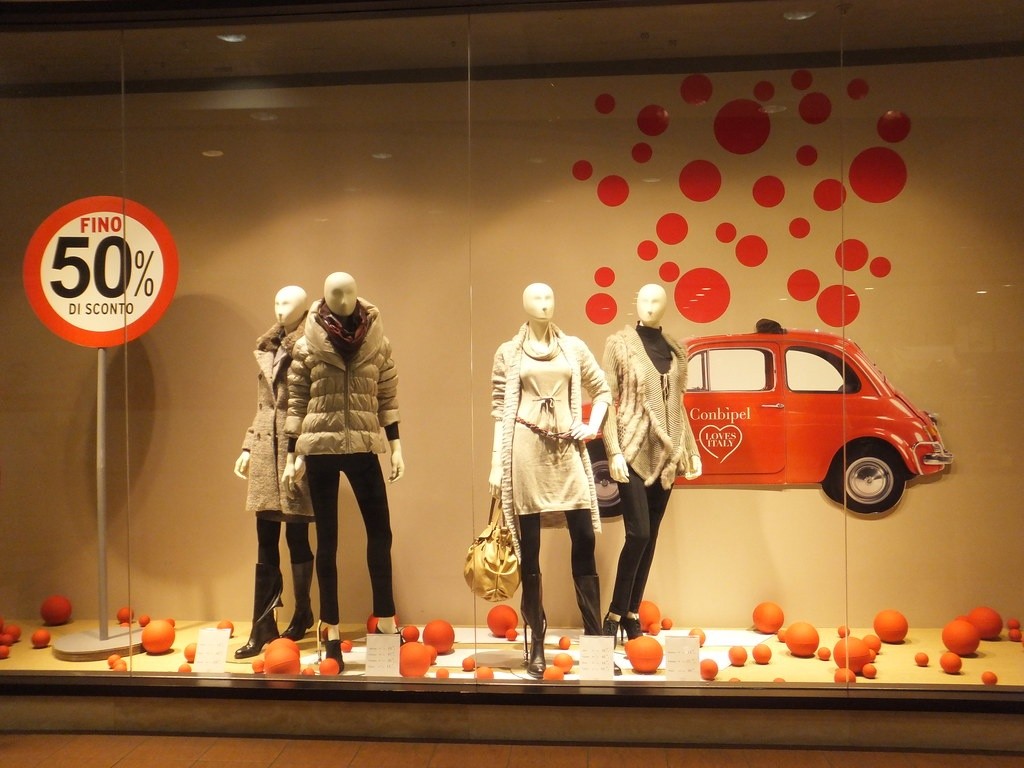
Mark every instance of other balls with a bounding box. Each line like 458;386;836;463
0;592;1024;688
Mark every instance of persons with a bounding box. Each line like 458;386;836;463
488;283;705;679
233;270;408;673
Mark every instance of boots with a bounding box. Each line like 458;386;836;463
235;563;280;659
282;558;314;641
574;574;622;676
521;573;546;678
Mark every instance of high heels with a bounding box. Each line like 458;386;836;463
325;637;344;671
375;624;405;647
620;616;643;642
603;612;620;649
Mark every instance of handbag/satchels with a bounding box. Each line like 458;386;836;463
464;497;522;603
